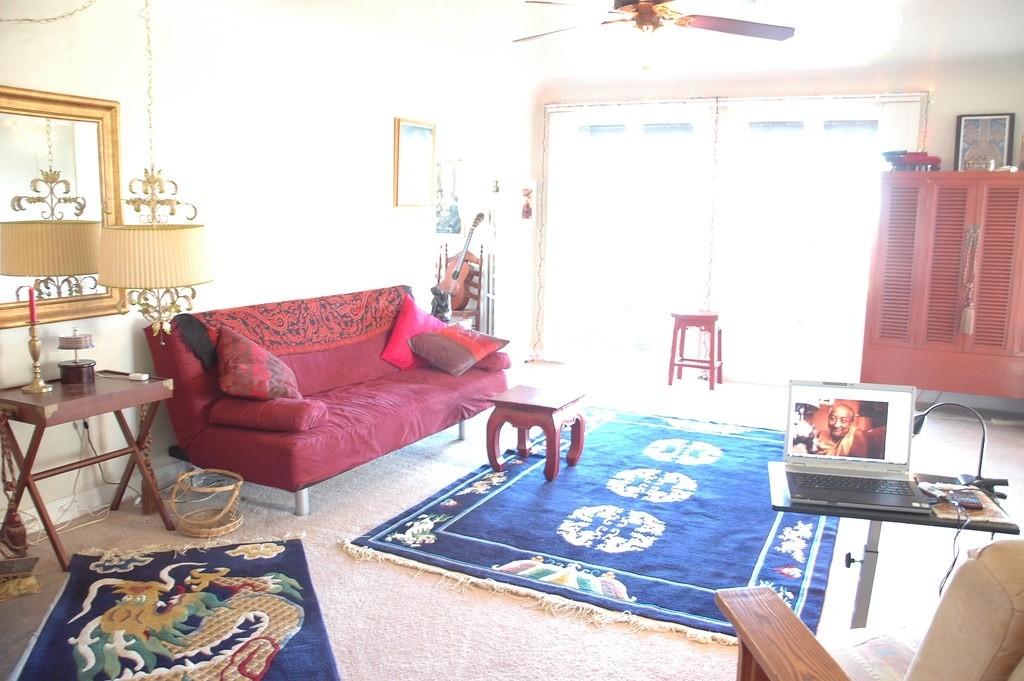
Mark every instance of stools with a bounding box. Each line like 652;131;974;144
667;312;724;391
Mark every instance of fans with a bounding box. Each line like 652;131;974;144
512;0;796;45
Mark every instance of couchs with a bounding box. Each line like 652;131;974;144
143;284;510;518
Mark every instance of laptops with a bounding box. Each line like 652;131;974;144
782;379;932;514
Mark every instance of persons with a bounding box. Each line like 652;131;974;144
814;403;869;458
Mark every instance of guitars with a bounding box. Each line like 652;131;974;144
438;213;484;311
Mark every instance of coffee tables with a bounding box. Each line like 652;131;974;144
486;385;585;482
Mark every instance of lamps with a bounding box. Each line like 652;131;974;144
0;116;102;303
96;0;215;350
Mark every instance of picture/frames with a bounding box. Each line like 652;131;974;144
953;113;1016;171
394;116;438;210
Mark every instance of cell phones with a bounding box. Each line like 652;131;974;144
950;491;984;509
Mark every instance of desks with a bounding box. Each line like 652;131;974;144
0;369;176;575
767;461;1020;629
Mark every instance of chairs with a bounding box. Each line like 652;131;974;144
435;241;483;332
713;539;1024;681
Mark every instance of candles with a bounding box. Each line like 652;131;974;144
28;284;36;323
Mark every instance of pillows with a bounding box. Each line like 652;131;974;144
405;322;512;378
217;326;303;401
380;293;451;372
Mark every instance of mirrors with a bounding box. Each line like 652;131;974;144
0;84;132;329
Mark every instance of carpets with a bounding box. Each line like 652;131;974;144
341;398;842;653
10;529;344;681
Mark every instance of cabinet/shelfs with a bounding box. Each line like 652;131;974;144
856;170;1024;400
